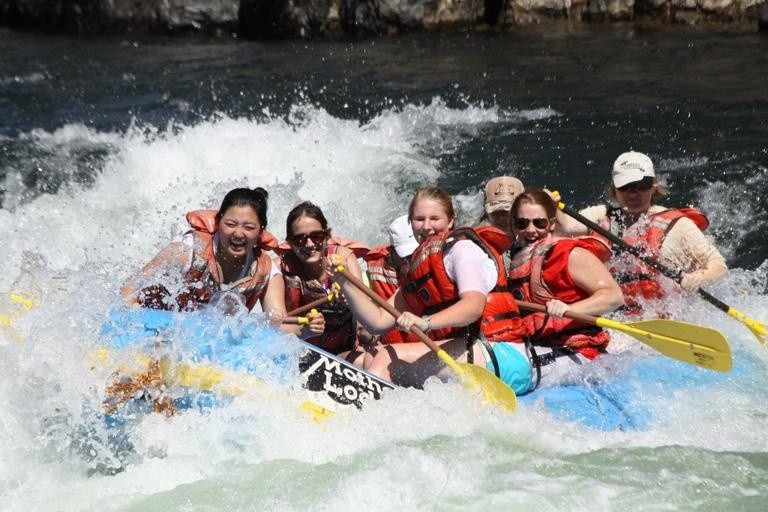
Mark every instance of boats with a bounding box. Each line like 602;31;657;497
0;290;768;477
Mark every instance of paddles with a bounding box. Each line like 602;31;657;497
333;260;516;413
544;189;768;350
512;299;731;371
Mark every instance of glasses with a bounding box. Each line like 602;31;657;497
617;178;654;192
287;229;329;248
513;215;551;229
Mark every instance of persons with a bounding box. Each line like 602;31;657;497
327;187;540;397
499;190;625;360
543;150;728;322
277;201;364;374
121;188;326;341
364;215;423;344
472;176;527;254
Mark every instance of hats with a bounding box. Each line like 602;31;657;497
485;176;525;213
389;214;421;258
612;152;655;188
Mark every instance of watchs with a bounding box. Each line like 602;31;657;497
423;313;433;335
